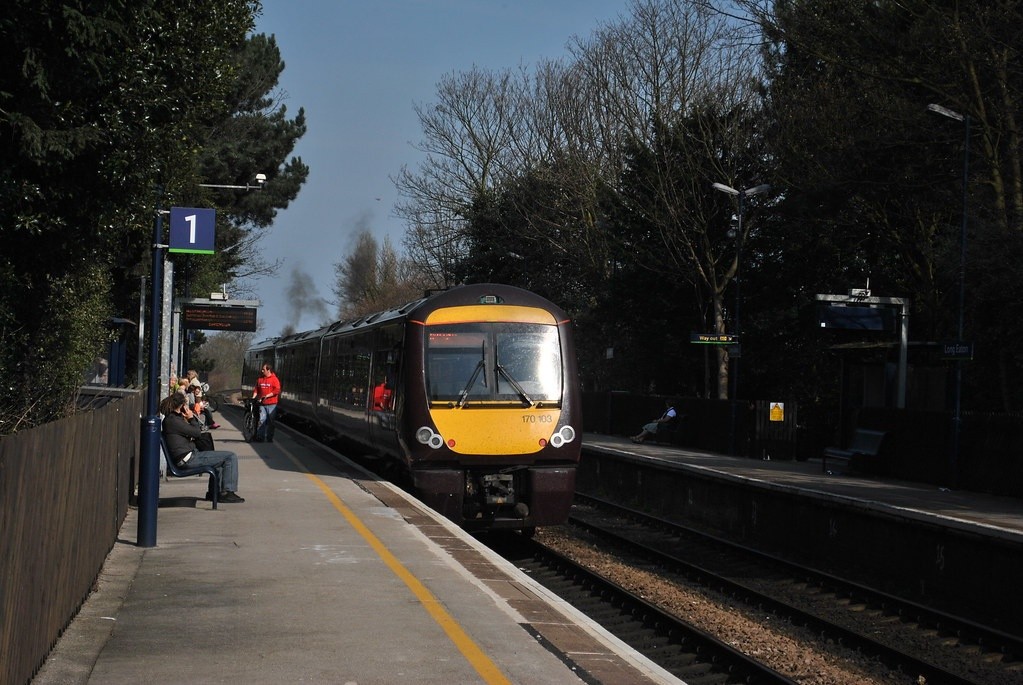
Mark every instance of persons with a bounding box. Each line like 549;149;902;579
160;393;245;503
252;362;281;442
630;400;676;443
170;369;220;429
373;375;393;410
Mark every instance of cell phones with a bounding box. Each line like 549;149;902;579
181;404;186;414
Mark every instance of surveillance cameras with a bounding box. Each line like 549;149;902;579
248;175;266;186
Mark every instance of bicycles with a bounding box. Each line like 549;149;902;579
201;382;218;412
237;397;265;443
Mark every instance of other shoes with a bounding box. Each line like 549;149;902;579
630;436;644;444
256;438;264;442
268;438;273;442
209;423;220;429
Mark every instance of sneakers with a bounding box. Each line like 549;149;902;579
218;491;245;503
206;492;214;501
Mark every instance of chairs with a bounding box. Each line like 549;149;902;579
160;431;218;510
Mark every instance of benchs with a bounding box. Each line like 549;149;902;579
823;428;892;478
646;414;687;447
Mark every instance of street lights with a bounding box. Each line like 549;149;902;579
710;181;743;452
924;100;973;489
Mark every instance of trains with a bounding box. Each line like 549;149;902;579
240;283;585;534
